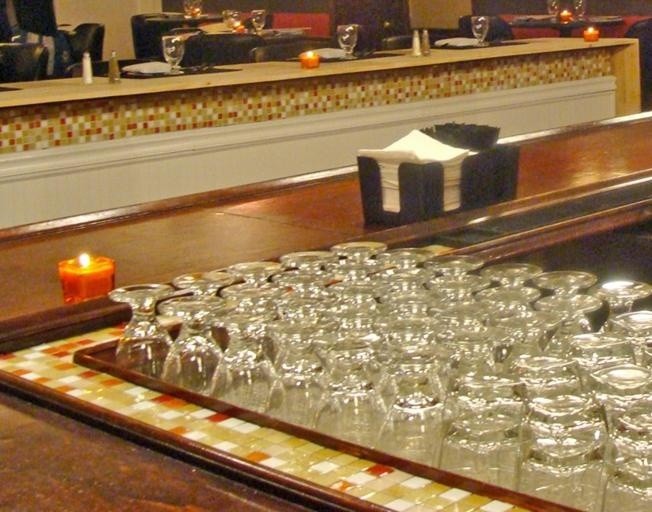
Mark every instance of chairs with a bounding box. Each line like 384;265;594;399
0;13;652;104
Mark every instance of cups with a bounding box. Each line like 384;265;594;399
182;0;202;19
223;9;243;30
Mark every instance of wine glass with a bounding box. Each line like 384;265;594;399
251;9;266;39
107;240;652;511
162;35;186;75
471;16;489;48
336;24;359;61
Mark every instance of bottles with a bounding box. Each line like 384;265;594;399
82;51;121;86
410;29;431;55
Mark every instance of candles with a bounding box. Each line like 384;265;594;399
60;250;116;304
